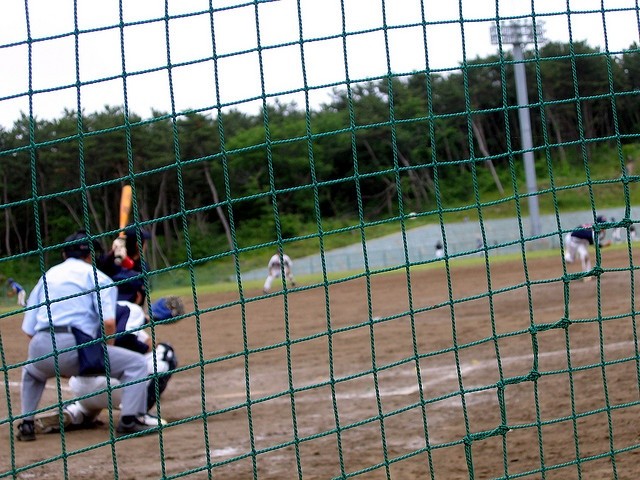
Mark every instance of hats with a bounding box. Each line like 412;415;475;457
597;216;607;222
126;223;151;240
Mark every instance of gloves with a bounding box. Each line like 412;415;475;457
114;247;127;259
112;238;126;252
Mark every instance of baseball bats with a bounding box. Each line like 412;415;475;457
114;185;133;266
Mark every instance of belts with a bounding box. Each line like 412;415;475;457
40;327;68;332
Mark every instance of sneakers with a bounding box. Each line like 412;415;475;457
34;409;75;434
16;420;35;440
137;414;167;425
117;415;157;434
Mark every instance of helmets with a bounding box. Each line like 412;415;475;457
112;268;146;307
64;230;104;270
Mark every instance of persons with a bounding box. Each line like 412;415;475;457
263;247;296;294
16;233;167;441
435;240;443;258
7;278;27;307
95;226;153;304
564;216;606;280
630;225;636;240
32;270;177;435
612;218;623;242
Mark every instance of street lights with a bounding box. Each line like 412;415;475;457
488;17;547;243
